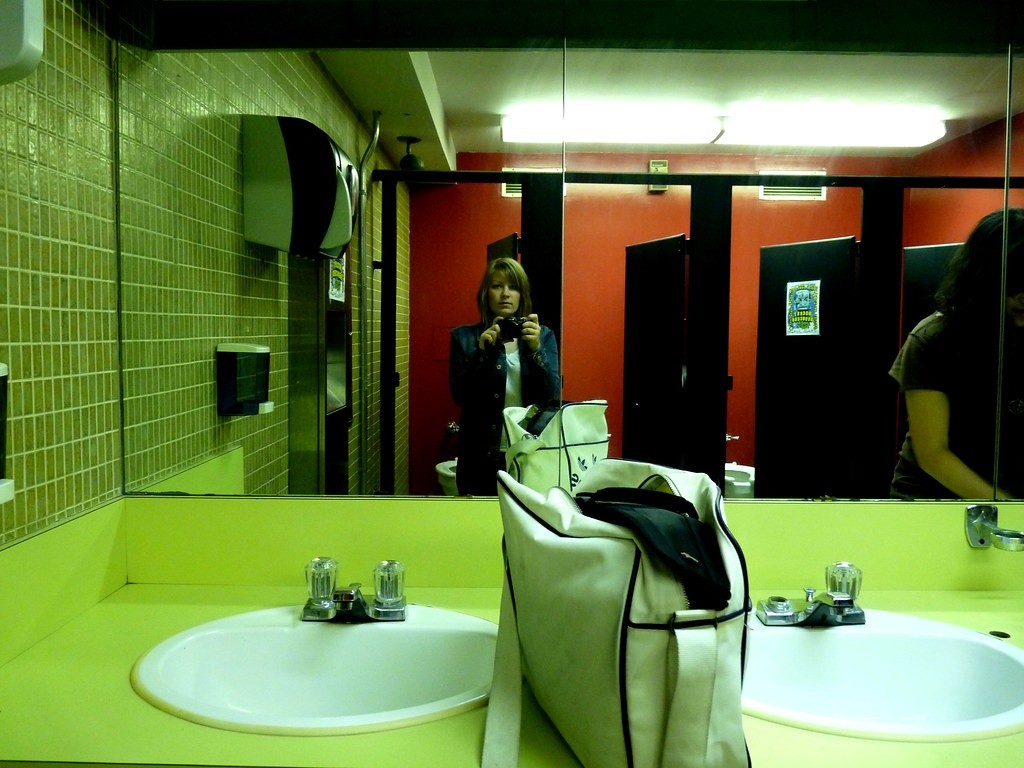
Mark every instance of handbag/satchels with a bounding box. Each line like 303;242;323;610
481;457;751;768
502;397;609;499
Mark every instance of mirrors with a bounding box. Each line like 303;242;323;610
113;38;1024;502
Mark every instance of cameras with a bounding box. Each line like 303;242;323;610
497;317;527;341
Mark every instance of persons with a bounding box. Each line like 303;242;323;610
887;207;1024;499
449;258;560;495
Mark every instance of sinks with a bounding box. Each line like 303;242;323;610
744;608;1024;744
129;603;498;736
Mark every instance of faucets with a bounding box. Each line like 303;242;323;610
299;554;409;627
752;560;867;629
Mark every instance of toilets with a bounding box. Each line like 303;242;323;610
725;461;754;496
435;456;460;496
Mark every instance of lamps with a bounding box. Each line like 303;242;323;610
396;136;424;170
